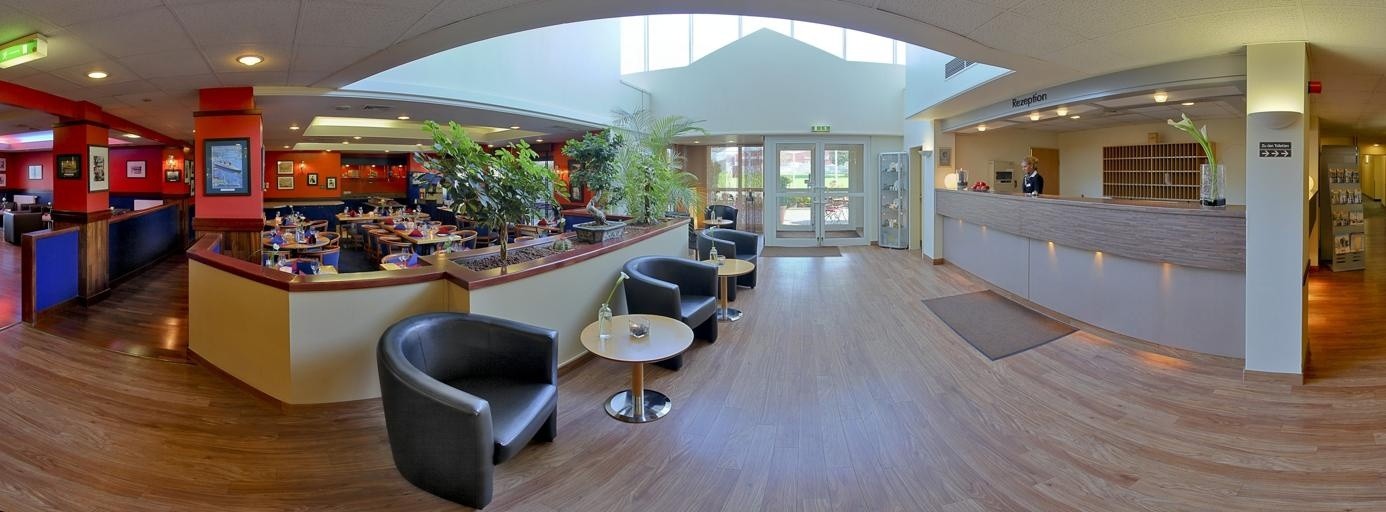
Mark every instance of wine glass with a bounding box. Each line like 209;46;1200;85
401;248;409;269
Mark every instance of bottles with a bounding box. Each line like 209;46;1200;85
711;210;715;222
599;303;613;339
710;246;717;264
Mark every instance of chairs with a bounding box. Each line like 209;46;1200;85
261;206;431;276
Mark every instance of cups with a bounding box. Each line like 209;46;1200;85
629;317;650;338
717;216;722;223
718;255;725;266
311;258;319;270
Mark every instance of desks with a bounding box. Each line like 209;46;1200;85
580;313;694;422
700;258;755;321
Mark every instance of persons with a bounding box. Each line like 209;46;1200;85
1020;155;1044;196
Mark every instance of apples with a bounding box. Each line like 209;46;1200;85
971;181;990;190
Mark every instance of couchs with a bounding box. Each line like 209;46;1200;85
623;256;719;371
378;311;559;507
696;228;758;302
700;205;738;231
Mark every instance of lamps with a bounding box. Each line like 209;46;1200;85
1249;111;1302;131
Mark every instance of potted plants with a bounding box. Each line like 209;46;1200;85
561;127;628;243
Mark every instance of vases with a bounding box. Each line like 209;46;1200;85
1199;162;1227;209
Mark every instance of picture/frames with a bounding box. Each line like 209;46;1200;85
0;136;337;198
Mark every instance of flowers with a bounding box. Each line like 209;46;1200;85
599;271;629;341
1167;112;1220;200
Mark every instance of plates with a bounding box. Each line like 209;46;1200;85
437;234;447;236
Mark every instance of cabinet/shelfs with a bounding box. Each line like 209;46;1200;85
1102;141;1218;201
879;151;909;249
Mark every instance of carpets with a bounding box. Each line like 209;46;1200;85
759;246;843;257
918;288;1080;361
